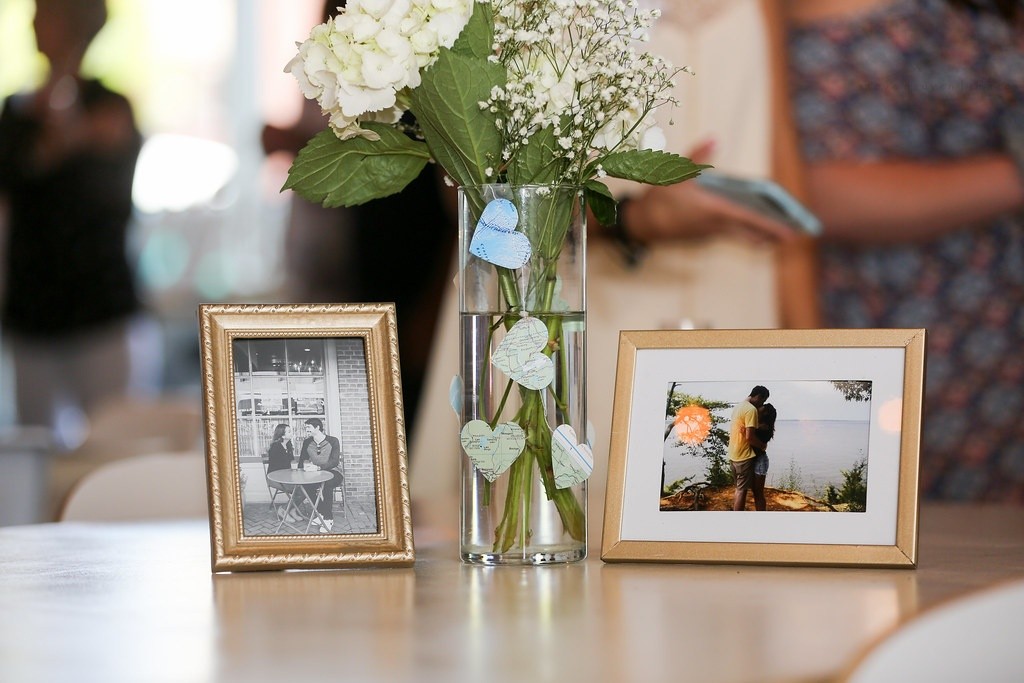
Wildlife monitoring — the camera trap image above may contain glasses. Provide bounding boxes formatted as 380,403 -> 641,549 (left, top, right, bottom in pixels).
317,444 -> 320,455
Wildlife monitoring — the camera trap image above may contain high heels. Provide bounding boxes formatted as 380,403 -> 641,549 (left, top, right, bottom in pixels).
277,506 -> 295,522
289,507 -> 303,521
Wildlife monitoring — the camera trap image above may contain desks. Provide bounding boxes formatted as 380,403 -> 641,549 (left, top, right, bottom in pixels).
0,448 -> 1024,683
266,469 -> 334,535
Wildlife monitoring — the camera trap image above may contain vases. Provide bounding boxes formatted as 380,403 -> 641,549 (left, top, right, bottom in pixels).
451,183 -> 591,573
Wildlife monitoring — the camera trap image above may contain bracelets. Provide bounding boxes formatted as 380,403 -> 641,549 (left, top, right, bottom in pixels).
603,196 -> 647,268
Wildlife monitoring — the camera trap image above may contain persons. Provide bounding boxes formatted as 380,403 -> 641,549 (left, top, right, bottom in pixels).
298,417 -> 340,533
728,386 -> 777,511
784,0 -> 1024,504
408,0 -> 822,501
267,424 -> 306,523
0,0 -> 143,430
260,0 -> 457,434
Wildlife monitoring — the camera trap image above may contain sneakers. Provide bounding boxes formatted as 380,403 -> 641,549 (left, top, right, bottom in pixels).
319,519 -> 333,533
311,513 -> 324,525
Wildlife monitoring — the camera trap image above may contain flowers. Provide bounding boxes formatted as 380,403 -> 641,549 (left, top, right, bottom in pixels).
280,0 -> 714,555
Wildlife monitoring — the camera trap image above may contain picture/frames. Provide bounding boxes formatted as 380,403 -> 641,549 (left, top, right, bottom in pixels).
600,329 -> 931,573
197,299 -> 417,573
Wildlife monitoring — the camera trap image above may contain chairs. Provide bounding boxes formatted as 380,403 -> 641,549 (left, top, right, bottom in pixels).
261,453 -> 307,522
329,452 -> 347,519
